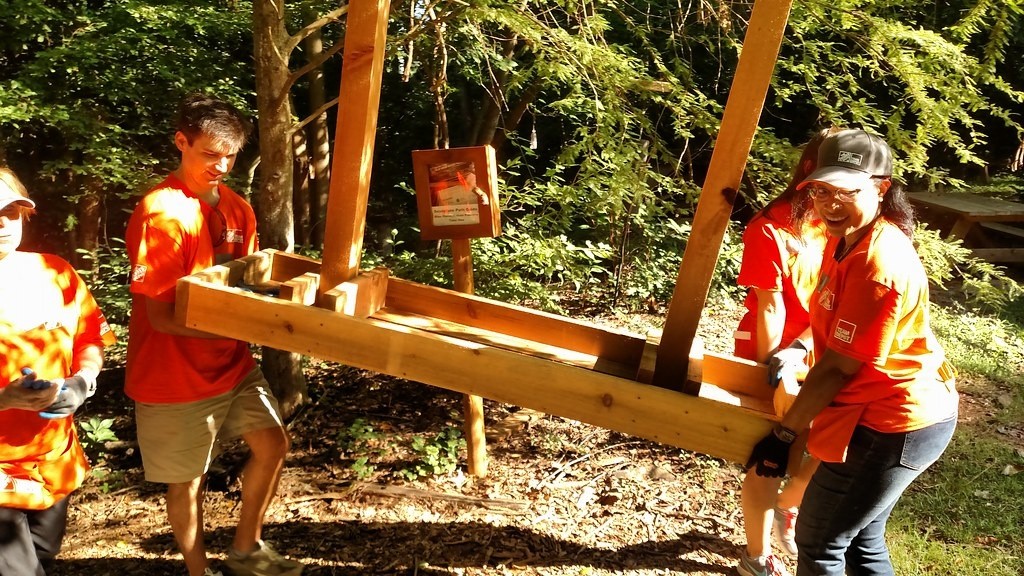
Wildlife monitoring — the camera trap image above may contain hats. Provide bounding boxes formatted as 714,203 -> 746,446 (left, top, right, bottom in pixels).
796,127 -> 895,191
0,177 -> 36,216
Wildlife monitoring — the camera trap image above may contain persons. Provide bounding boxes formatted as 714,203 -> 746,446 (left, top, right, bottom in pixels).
0,167 -> 119,576
123,92 -> 310,576
744,127 -> 959,576
733,127 -> 862,576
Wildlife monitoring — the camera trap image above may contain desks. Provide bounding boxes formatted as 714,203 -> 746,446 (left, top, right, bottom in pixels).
905,192 -> 1024,262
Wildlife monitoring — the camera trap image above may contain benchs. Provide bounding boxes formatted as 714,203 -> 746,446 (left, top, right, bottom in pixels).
981,221 -> 1024,237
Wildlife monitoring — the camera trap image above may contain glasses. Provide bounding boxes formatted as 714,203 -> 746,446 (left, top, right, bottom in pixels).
806,185 -> 876,202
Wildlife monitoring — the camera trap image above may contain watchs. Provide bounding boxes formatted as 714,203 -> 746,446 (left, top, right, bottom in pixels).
774,424 -> 797,444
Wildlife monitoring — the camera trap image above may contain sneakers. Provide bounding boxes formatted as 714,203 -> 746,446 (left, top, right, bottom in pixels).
226,539 -> 306,576
773,506 -> 798,558
737,547 -> 794,576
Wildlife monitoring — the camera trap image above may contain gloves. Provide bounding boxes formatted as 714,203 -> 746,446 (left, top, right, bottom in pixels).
765,338 -> 809,389
39,372 -> 91,419
20,368 -> 66,391
745,423 -> 799,480
